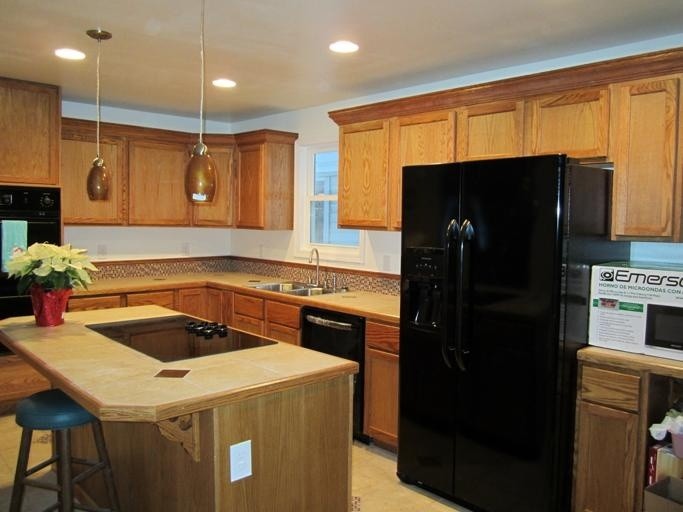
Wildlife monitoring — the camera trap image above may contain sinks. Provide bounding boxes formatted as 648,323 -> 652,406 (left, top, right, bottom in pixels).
256,283 -> 306,292
283,289 -> 331,296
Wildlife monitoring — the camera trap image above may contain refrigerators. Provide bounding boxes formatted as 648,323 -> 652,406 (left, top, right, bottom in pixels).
395,153 -> 614,512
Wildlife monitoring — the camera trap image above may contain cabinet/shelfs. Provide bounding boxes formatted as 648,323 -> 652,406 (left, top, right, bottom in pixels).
327,87 -> 457,232
364,311 -> 400,456
61,117 -> 194,227
235,287 -> 301,346
570,346 -> 683,512
0,75 -> 61,188
176,283 -> 206,318
611,47 -> 683,243
193,129 -> 298,230
458,59 -> 611,164
206,282 -> 234,328
66,284 -> 175,312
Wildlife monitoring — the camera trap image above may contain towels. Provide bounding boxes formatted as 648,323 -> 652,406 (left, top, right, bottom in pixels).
1,220 -> 28,273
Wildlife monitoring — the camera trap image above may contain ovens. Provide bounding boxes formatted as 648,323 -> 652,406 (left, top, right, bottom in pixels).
0,185 -> 61,357
298,306 -> 364,444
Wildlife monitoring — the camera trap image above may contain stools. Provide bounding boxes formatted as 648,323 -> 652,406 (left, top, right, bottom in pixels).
10,388 -> 120,512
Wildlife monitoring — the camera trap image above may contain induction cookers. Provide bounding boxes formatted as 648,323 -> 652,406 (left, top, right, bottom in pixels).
86,313 -> 278,364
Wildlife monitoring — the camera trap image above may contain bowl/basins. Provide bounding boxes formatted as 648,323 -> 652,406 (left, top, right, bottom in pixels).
669,432 -> 683,459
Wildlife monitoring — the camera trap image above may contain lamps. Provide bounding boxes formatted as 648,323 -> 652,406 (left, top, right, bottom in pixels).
86,28 -> 111,201
183,4 -> 219,204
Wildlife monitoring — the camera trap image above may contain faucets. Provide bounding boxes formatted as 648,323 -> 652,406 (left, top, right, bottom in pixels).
309,247 -> 319,285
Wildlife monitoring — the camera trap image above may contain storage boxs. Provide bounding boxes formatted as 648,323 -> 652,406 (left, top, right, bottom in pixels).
644,475 -> 683,512
588,261 -> 683,361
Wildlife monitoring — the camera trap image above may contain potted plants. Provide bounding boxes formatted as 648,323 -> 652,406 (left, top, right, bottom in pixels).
7,240 -> 100,327
